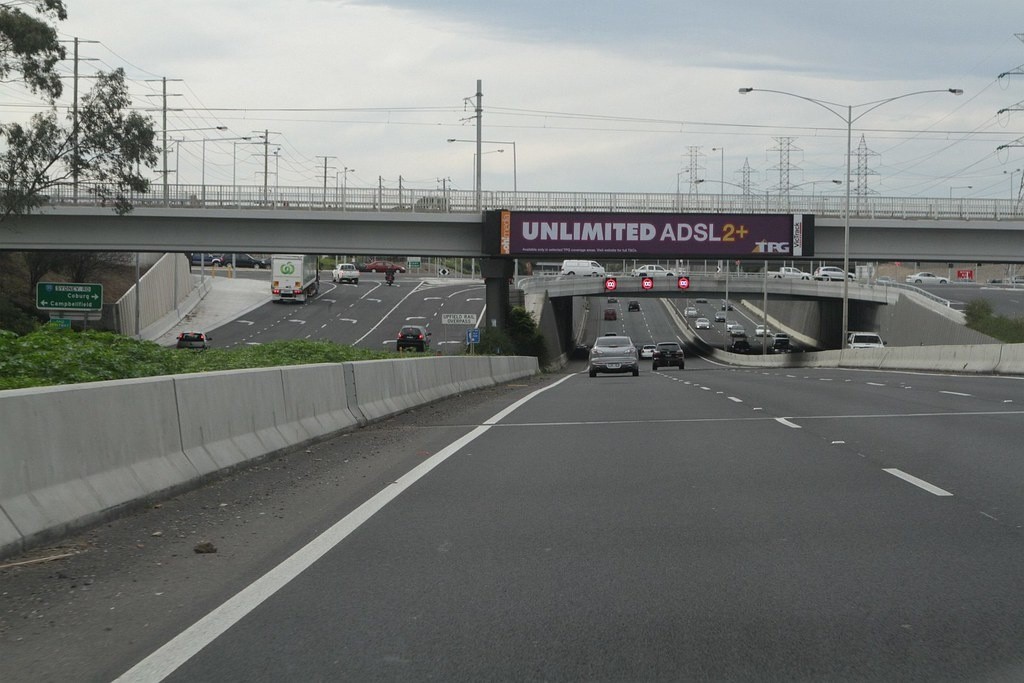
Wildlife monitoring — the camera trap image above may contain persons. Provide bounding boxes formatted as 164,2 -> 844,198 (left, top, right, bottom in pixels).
385,266 -> 394,283
